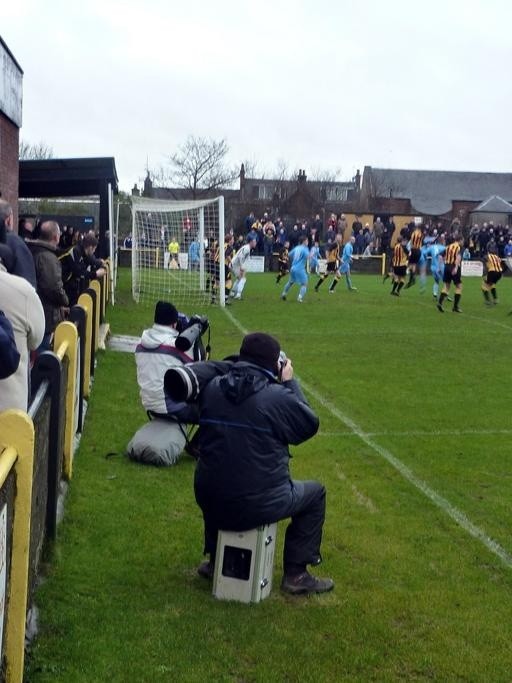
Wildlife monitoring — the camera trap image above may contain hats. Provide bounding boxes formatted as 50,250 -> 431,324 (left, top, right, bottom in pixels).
154,300 -> 179,325
239,332 -> 280,376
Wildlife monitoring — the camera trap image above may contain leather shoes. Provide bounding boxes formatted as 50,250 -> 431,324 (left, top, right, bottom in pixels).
184,441 -> 200,459
197,559 -> 214,579
279,569 -> 335,594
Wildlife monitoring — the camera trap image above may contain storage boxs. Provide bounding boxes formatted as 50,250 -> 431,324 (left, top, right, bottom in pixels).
212,523 -> 277,604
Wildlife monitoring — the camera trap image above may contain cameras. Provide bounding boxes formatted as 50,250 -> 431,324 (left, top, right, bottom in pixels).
276,351 -> 287,378
174,312 -> 209,351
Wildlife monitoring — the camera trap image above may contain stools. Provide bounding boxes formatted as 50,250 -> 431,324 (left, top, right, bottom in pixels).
146,409 -> 196,445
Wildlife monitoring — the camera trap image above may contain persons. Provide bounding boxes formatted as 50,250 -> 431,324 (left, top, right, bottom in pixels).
135,302 -> 203,457
125,203 -> 511,315
0,201 -> 107,433
191,332 -> 335,596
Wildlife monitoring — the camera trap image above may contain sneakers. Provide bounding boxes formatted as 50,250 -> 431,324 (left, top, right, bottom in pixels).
391,290 -> 400,296
282,293 -> 287,301
436,302 -> 444,312
297,294 -> 304,303
447,296 -> 453,302
453,307 -> 463,313
350,286 -> 357,291
432,293 -> 438,303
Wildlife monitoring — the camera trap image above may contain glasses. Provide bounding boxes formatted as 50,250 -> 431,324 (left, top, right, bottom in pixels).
57,231 -> 62,235
